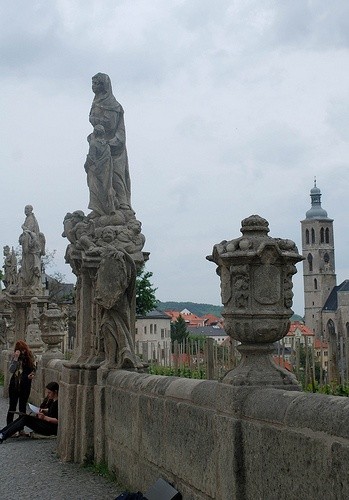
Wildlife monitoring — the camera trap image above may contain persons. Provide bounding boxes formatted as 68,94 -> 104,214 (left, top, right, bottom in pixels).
87,73 -> 131,209
0,382 -> 59,444
5,342 -> 36,438
21,205 -> 40,276
62,211 -> 95,250
97,227 -> 116,248
85,125 -> 113,219
2,246 -> 14,284
121,216 -> 141,240
